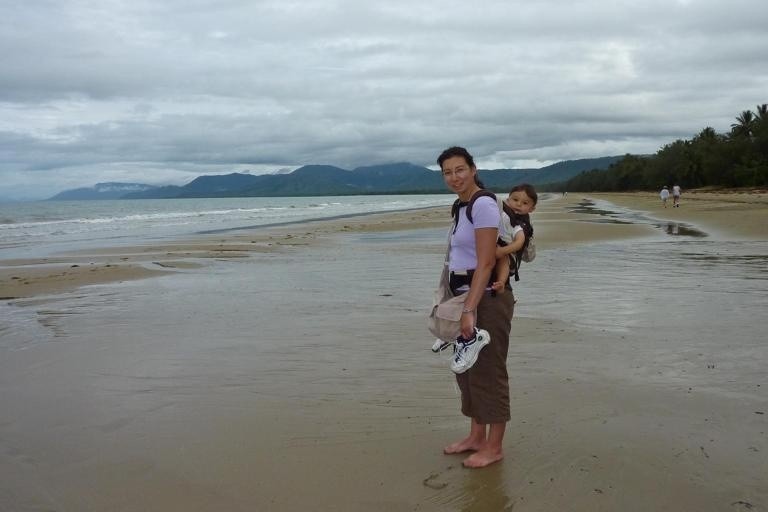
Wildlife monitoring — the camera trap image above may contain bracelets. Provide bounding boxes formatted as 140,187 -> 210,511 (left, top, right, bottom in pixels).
462,308 -> 475,313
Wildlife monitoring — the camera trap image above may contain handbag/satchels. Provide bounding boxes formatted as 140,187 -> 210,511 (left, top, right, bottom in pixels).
427,265 -> 477,342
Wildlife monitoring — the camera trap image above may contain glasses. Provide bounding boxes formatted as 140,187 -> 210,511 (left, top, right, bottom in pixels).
443,165 -> 470,177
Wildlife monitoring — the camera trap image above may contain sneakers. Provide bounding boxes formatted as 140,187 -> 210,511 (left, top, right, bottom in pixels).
448,326 -> 491,375
431,339 -> 452,353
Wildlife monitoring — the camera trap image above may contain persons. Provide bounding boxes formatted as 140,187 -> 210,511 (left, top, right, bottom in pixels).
672,182 -> 681,208
659,185 -> 669,209
491,183 -> 537,295
434,146 -> 517,471
